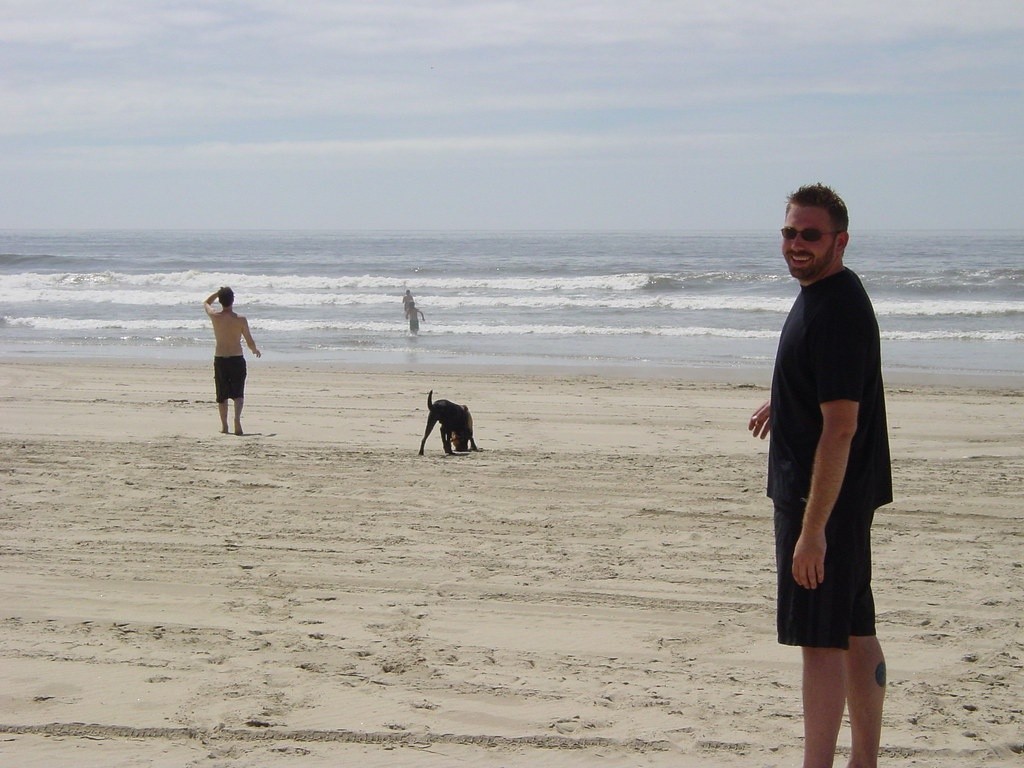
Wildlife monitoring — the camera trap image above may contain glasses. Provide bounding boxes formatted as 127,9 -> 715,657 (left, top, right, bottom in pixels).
780,226 -> 835,242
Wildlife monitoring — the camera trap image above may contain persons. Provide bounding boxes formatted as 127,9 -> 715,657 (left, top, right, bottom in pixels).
745,183 -> 895,768
402,289 -> 414,319
405,301 -> 425,335
201,287 -> 262,435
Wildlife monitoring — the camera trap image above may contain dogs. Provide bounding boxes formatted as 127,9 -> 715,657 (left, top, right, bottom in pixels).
417,388 -> 481,459
